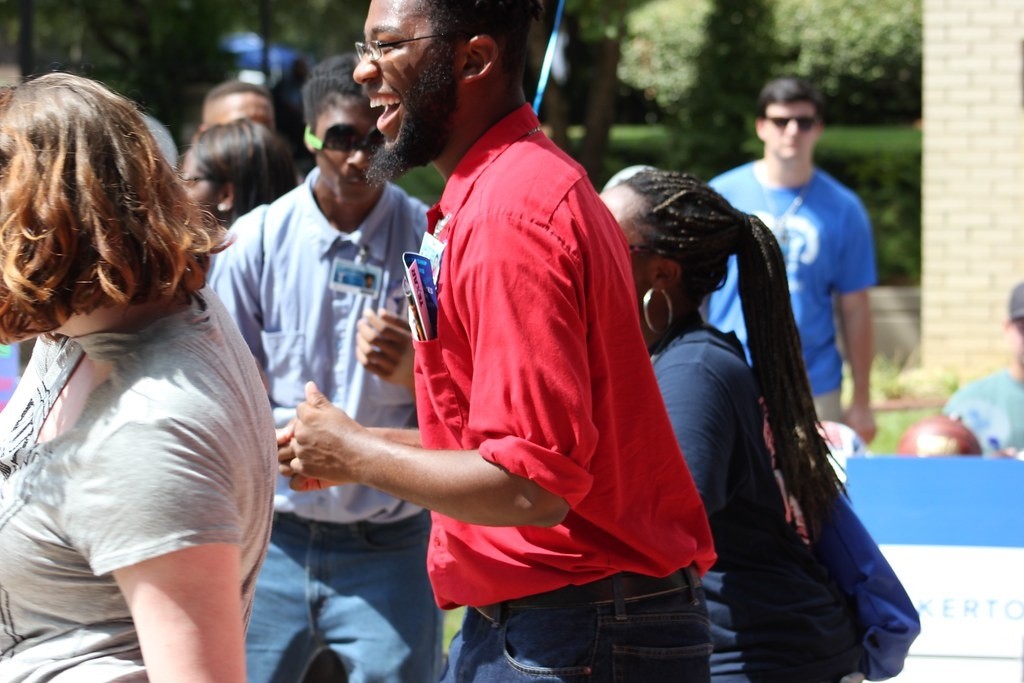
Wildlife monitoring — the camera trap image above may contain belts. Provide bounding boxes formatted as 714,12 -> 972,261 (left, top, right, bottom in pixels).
470,563 -> 704,623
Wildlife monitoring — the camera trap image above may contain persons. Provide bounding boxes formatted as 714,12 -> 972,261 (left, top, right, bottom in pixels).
278,0 -> 717,681
198,82 -> 275,131
946,278 -> 1023,459
594,169 -> 922,683
180,120 -> 298,228
700,80 -> 878,454
2,73 -> 277,681
209,52 -> 442,682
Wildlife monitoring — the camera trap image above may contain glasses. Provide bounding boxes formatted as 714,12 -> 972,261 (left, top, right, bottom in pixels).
355,30 -> 471,61
178,175 -> 213,187
764,115 -> 820,132
304,124 -> 385,155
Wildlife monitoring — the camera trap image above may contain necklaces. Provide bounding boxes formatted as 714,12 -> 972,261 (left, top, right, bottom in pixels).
758,160 -> 812,228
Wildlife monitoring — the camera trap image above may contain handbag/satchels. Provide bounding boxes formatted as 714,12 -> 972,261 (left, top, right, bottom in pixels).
728,331 -> 922,682
